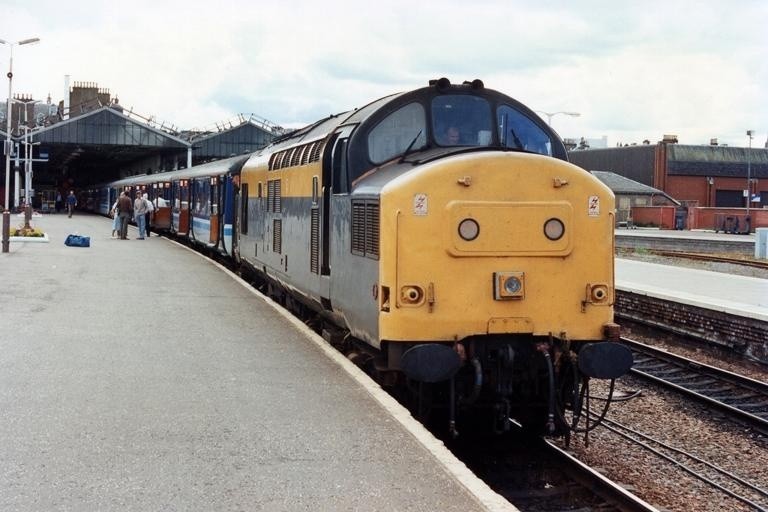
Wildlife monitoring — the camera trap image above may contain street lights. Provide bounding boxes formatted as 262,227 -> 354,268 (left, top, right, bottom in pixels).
532,107 -> 582,126
745,129 -> 753,215
0,35 -> 40,256
9,94 -> 46,239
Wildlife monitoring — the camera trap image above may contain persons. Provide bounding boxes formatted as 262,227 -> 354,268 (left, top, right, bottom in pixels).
111,191 -> 160,240
66,190 -> 78,218
152,193 -> 166,207
56,191 -> 63,213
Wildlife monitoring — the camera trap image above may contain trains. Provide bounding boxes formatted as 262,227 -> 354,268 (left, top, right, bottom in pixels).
78,73 -> 634,469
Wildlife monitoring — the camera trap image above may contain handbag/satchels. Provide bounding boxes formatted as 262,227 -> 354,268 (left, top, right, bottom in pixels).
64,234 -> 91,247
